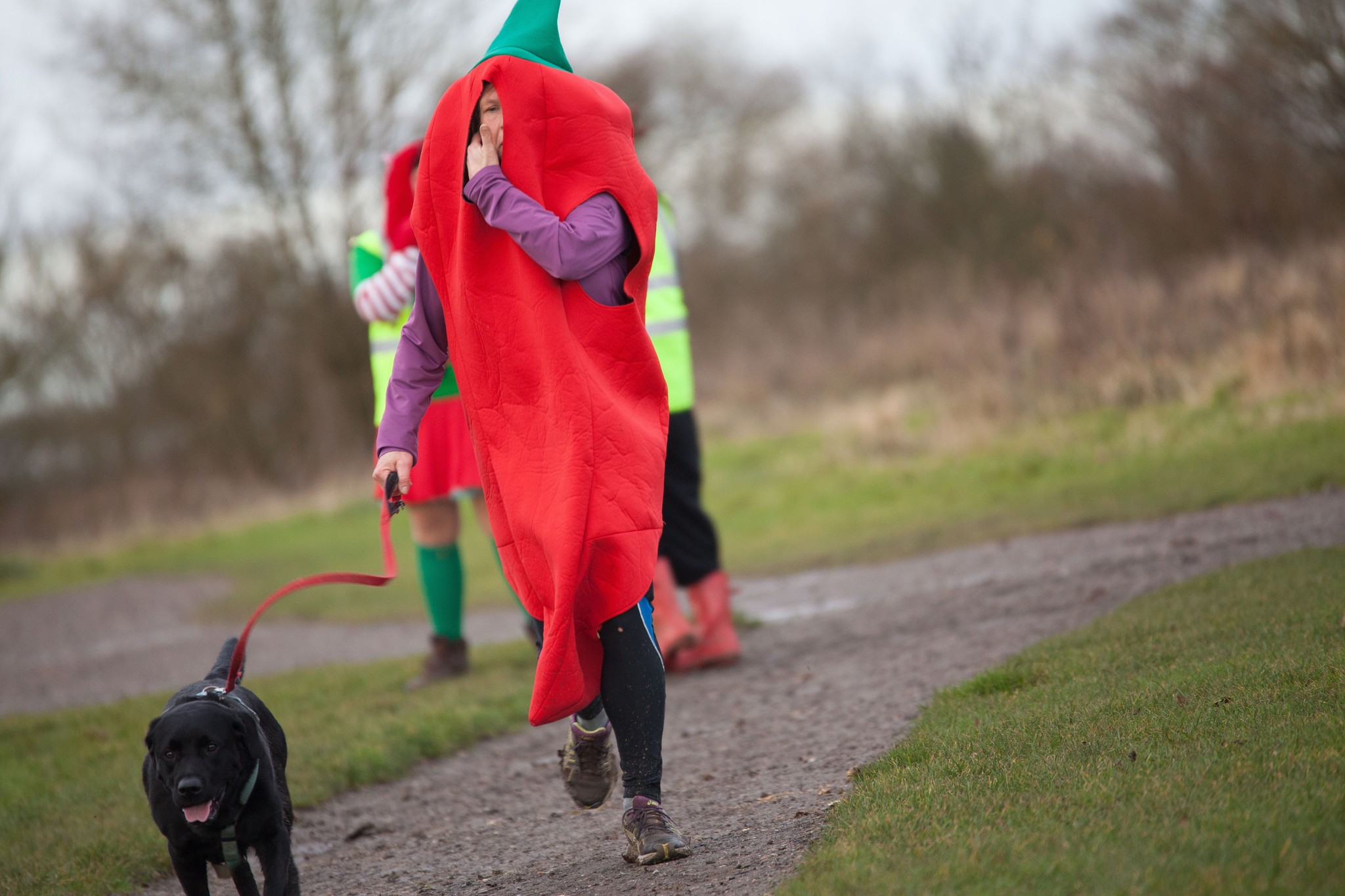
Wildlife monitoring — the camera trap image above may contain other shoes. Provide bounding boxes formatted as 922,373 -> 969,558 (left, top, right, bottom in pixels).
405,634 -> 469,694
649,555 -> 741,672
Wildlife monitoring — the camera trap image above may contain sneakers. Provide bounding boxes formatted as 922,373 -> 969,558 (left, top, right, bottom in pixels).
557,712 -> 619,810
621,795 -> 692,866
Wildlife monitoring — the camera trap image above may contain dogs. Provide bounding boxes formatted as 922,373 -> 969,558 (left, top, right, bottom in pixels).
142,638 -> 301,896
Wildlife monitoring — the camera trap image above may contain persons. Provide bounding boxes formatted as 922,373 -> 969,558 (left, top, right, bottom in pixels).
629,186 -> 741,679
368,63 -> 694,871
345,137 -> 562,678
